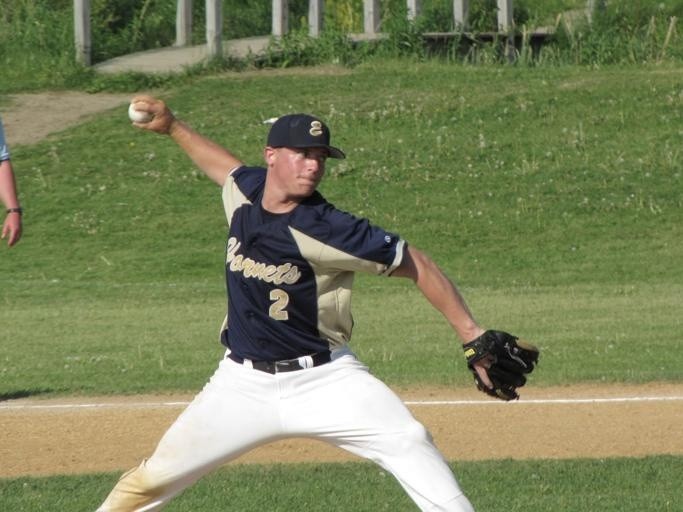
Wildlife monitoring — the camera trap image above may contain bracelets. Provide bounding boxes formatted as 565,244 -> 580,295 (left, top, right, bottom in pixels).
4,207 -> 22,217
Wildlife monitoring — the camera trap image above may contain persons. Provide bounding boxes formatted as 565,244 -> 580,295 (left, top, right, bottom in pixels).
93,92 -> 538,511
0,114 -> 22,249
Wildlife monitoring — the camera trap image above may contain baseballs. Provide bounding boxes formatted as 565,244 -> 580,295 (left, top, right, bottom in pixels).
128,99 -> 152,123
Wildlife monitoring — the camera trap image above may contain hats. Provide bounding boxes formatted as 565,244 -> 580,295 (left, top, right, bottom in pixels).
264,113 -> 347,163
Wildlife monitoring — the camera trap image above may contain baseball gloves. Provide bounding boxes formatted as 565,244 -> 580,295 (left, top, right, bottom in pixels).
462,331 -> 539,400
